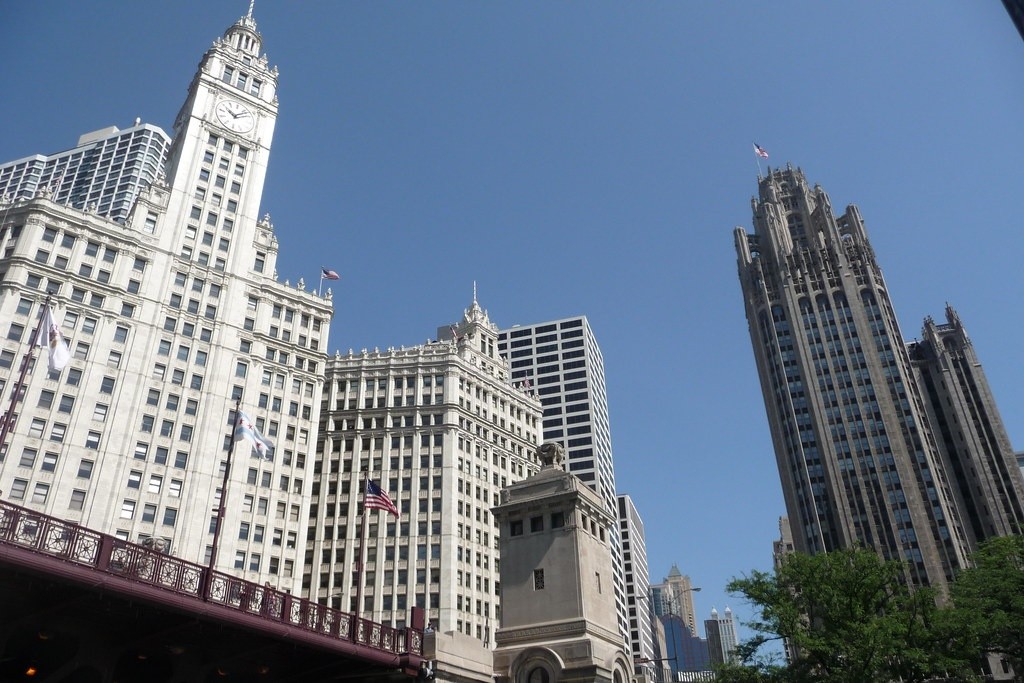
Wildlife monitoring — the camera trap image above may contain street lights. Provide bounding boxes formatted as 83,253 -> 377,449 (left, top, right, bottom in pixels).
665,588 -> 703,671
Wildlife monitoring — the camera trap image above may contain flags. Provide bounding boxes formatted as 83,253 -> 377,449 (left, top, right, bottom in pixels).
754,142 -> 768,158
57,169 -> 63,185
234,409 -> 273,459
450,327 -> 456,337
321,268 -> 340,280
36,304 -> 71,373
365,477 -> 399,519
525,379 -> 530,389
